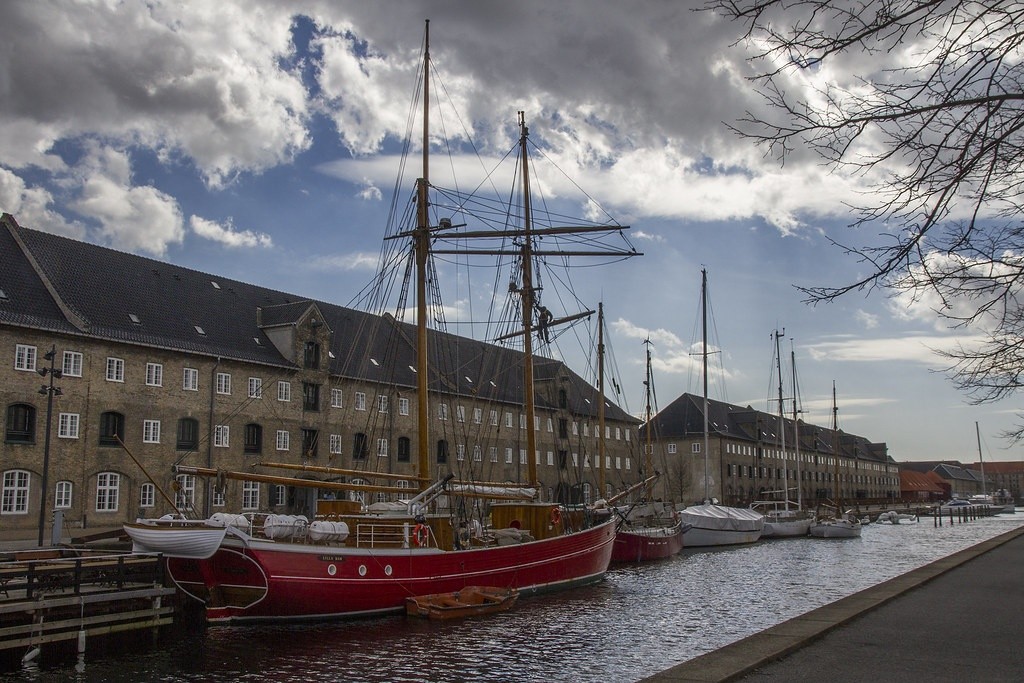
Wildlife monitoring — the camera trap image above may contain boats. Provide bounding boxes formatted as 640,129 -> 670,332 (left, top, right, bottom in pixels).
405,585 -> 521,621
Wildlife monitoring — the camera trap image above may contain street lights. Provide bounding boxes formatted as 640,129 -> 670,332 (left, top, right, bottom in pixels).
37,343 -> 62,546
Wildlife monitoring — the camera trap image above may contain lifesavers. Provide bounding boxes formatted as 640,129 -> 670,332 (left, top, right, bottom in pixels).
412,523 -> 428,546
551,508 -> 561,524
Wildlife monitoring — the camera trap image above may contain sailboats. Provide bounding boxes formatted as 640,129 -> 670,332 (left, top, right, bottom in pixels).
928,422 -> 1006,515
119,16 -> 618,622
747,327 -> 817,536
810,379 -> 862,538
676,269 -> 763,548
610,337 -> 684,560
